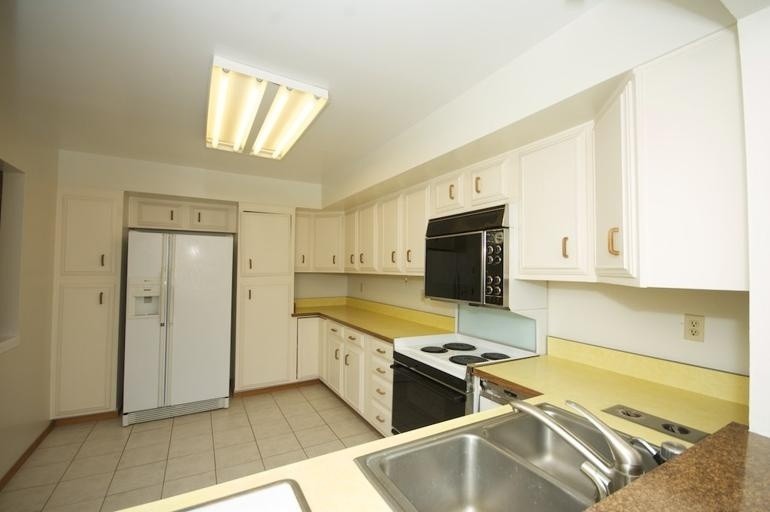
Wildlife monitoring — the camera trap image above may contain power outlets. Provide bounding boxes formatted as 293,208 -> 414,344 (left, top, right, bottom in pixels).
684,314 -> 705,342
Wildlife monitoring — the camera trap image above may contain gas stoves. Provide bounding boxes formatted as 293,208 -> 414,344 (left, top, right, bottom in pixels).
392,335 -> 540,395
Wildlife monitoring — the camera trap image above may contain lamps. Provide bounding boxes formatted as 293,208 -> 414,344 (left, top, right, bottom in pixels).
205,56 -> 329,161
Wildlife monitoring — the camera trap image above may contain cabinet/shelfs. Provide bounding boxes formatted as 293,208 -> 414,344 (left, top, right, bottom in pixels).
240,207 -> 292,277
58,278 -> 117,413
125,192 -> 238,235
235,277 -> 293,392
323,319 -> 394,437
294,210 -> 315,273
521,119 -> 596,283
57,193 -> 118,277
596,81 -> 641,287
378,180 -> 427,277
430,149 -> 511,216
344,201 -> 378,274
315,210 -> 344,273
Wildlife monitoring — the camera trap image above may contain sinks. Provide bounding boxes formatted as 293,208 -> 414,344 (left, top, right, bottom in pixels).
484,401 -> 676,503
353,431 -> 590,512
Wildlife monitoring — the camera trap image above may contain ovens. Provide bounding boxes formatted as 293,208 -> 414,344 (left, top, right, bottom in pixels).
390,358 -> 466,437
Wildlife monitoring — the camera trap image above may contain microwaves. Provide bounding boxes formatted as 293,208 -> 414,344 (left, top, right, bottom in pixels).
423,228 -> 509,309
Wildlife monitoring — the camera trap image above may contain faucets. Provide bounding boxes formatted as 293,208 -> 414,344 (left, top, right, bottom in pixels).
629,437 -> 685,467
508,399 -> 644,504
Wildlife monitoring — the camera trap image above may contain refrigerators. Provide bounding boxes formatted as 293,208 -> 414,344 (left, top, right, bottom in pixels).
119,227 -> 234,429
